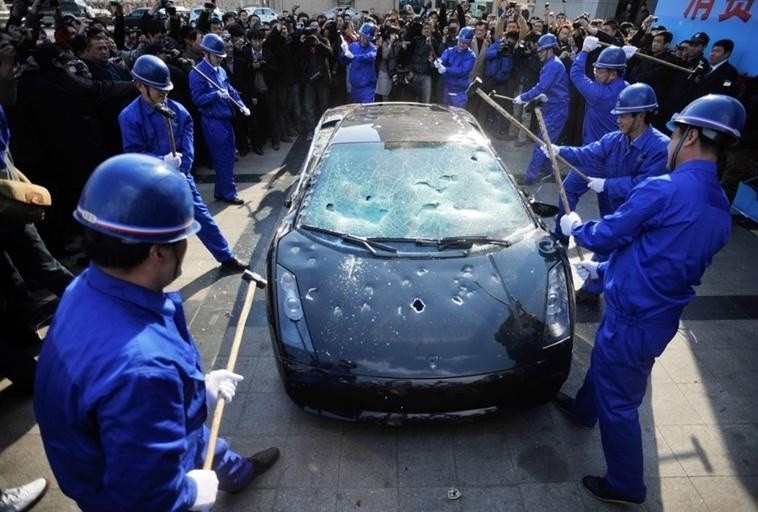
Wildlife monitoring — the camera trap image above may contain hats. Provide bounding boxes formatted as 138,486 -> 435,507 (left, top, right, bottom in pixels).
649,25 -> 667,31
691,31 -> 710,42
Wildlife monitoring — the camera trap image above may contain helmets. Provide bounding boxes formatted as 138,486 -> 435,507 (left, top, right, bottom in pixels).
129,53 -> 174,94
591,46 -> 628,69
534,32 -> 558,52
666,93 -> 746,139
455,26 -> 475,44
73,150 -> 202,246
607,83 -> 659,117
199,33 -> 228,60
358,21 -> 376,40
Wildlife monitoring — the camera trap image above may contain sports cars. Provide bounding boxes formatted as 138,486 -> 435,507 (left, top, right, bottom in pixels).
259,94 -> 579,441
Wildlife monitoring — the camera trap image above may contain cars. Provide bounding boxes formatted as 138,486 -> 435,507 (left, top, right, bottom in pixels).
320,4 -> 361,21
0,0 -> 282,32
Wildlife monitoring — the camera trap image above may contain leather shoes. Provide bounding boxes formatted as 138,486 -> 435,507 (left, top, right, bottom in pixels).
240,137 -> 316,157
214,192 -> 246,208
226,445 -> 280,496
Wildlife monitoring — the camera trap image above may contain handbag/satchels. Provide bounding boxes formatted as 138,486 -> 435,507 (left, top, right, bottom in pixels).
0,177 -> 52,223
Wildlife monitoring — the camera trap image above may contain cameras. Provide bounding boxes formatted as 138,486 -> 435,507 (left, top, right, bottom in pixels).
278,16 -> 290,22
71,62 -> 85,71
519,43 -> 531,53
158,0 -> 177,16
503,44 -> 513,53
509,2 -> 516,7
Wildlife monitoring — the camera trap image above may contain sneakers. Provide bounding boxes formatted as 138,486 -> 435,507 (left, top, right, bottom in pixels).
513,139 -> 527,148
551,391 -> 597,431
218,260 -> 252,271
517,179 -> 537,186
581,475 -> 647,507
541,173 -> 555,183
0,478 -> 49,512
573,287 -> 601,305
496,133 -> 516,140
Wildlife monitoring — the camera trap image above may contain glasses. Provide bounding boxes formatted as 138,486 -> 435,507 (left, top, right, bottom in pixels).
593,68 -> 612,74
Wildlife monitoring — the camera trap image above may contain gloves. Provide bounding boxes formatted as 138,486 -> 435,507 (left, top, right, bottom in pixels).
573,260 -> 600,282
512,95 -> 523,106
182,468 -> 219,512
433,59 -> 447,75
340,42 -> 353,61
239,105 -> 252,119
558,211 -> 583,238
587,176 -> 606,195
164,149 -> 184,169
540,141 -> 560,162
216,88 -> 230,101
204,367 -> 245,408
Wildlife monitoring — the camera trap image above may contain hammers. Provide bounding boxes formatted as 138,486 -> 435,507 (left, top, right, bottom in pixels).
154,102 -> 176,157
597,41 -> 704,81
465,76 -> 592,182
202,268 -> 268,470
488,90 -> 528,103
523,94 -> 591,283
425,37 -> 440,63
178,57 -> 244,113
337,30 -> 348,53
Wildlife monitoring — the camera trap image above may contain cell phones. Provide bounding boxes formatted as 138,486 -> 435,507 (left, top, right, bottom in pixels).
205,3 -> 216,9
477,4 -> 485,10
650,16 -> 659,23
